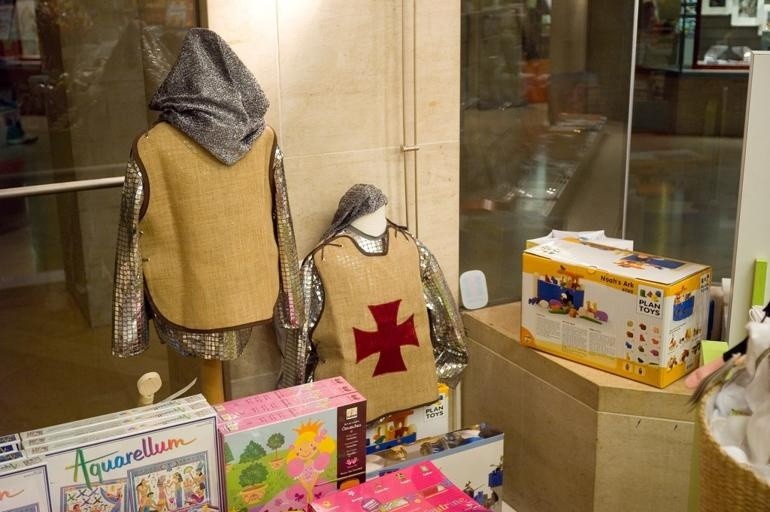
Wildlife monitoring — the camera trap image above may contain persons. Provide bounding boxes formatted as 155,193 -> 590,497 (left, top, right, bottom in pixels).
113,29 -> 303,361
276,183 -> 470,428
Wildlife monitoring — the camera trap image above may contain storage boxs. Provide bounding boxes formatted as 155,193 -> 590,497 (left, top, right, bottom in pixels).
520,229 -> 712,389
369,381 -> 449,450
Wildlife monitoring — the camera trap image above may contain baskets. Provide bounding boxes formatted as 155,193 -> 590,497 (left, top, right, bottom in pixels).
691,360 -> 770,512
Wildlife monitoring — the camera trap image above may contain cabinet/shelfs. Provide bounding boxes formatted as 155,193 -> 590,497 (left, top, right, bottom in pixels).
632,62 -> 754,136
467,299 -> 720,512
